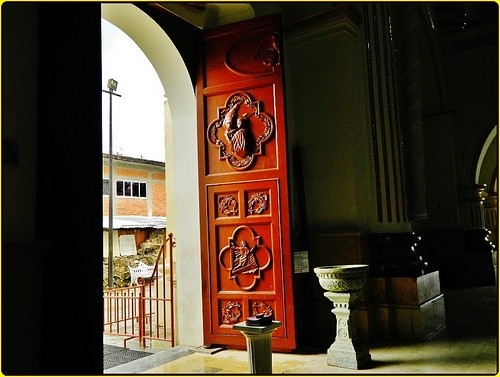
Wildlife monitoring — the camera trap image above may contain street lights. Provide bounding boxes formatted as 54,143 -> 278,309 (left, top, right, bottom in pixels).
102,78 -> 120,289
